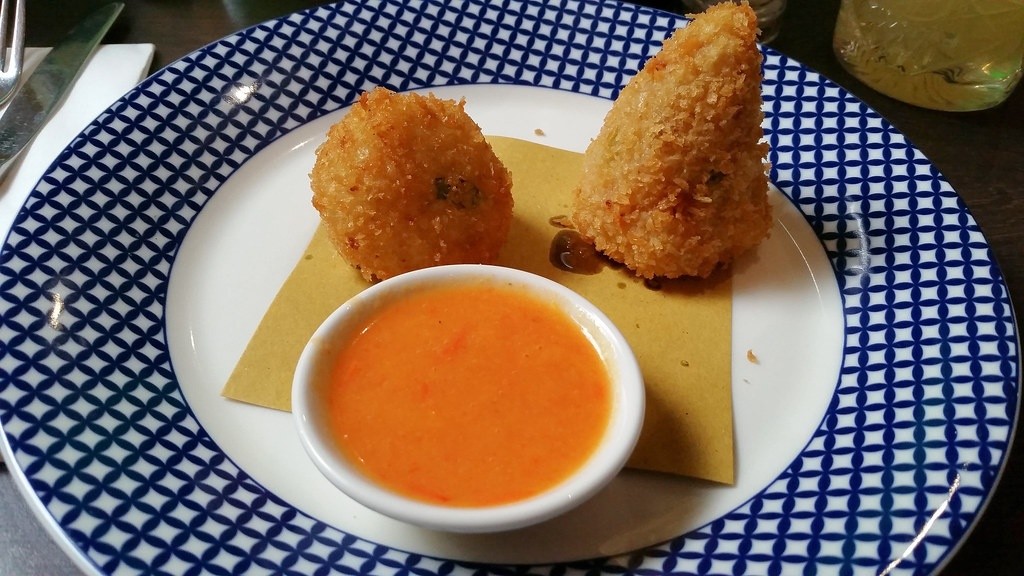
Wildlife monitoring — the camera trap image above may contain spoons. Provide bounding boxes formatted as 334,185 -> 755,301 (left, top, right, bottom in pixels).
0,0 -> 26,107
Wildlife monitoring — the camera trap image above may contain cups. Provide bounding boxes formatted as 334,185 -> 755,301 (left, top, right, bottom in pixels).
696,0 -> 786,46
833,0 -> 1024,113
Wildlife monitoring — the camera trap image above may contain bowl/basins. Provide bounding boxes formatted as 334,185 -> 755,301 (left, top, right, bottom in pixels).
290,263 -> 646,536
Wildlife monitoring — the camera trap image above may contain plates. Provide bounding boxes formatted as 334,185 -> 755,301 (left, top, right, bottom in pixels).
0,0 -> 1024,576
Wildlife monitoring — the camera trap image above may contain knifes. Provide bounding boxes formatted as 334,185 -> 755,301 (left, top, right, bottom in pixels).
0,0 -> 125,186
